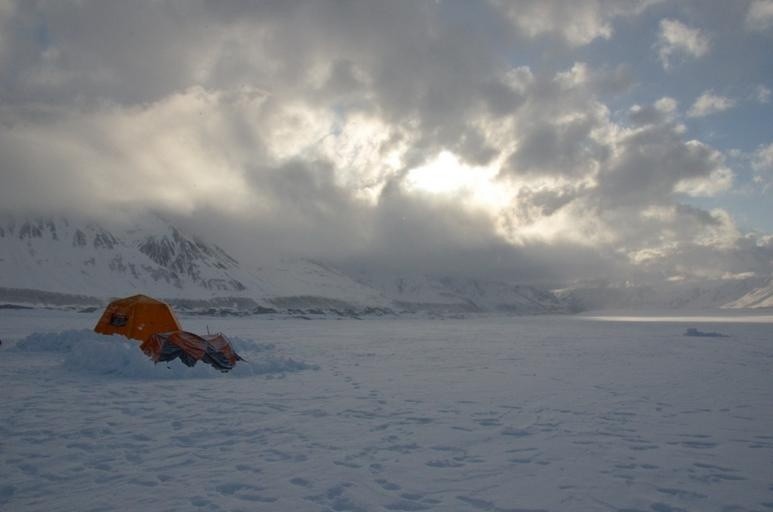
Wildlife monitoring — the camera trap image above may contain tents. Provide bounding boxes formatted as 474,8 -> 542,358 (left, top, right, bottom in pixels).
95,294 -> 182,343
139,329 -> 249,374
